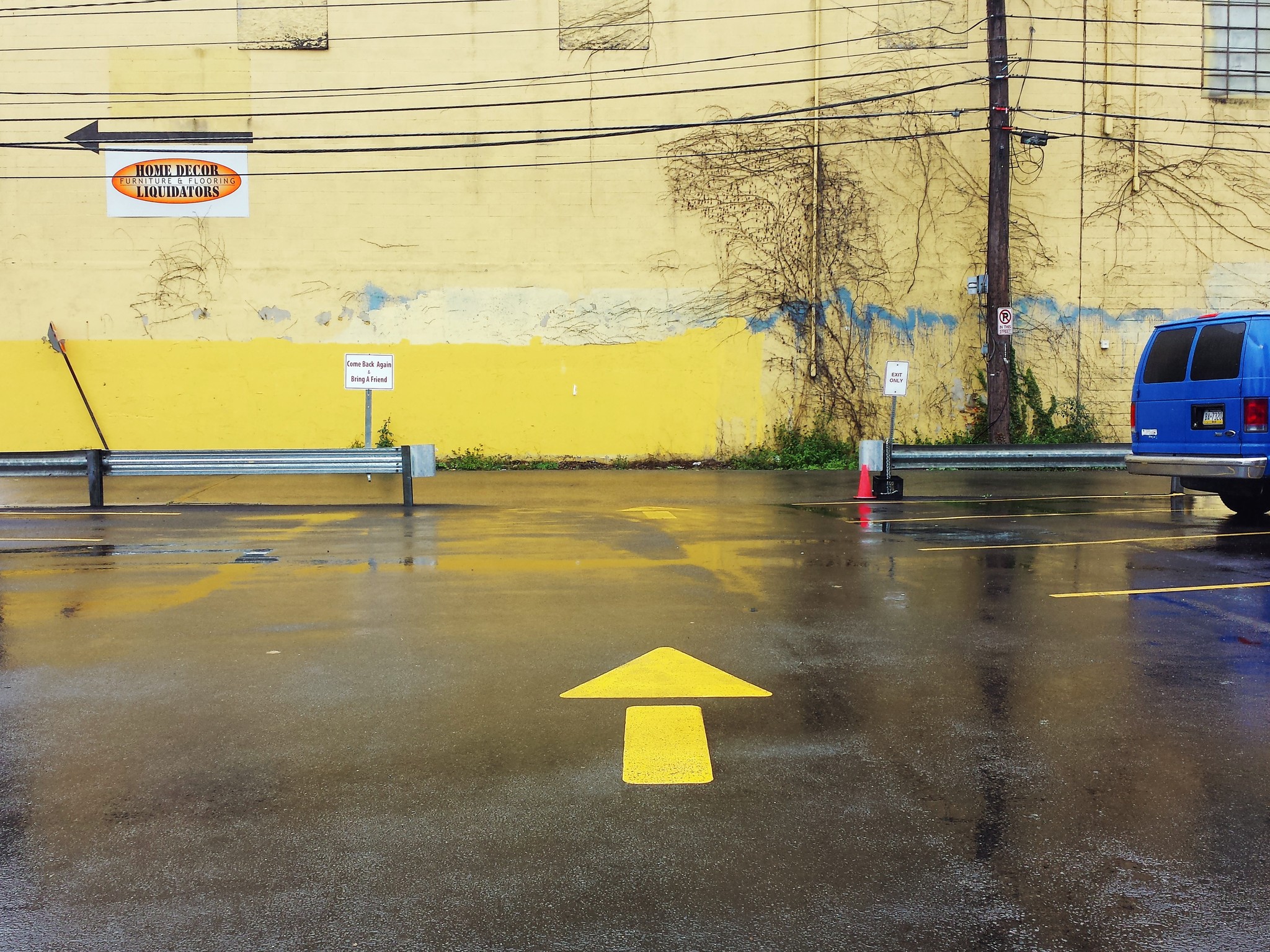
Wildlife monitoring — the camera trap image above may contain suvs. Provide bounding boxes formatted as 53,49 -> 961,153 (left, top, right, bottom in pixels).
1124,309 -> 1269,526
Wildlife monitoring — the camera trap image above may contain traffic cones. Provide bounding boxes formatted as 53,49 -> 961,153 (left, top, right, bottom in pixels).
852,464 -> 876,499
